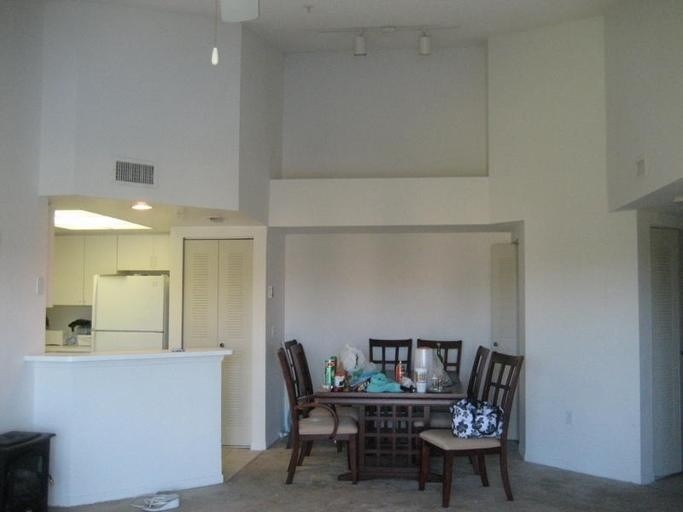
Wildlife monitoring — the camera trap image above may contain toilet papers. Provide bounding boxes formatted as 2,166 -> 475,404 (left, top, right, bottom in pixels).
415,347 -> 442,388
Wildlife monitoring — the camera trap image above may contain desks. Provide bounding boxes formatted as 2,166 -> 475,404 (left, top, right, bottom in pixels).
314,370 -> 469,481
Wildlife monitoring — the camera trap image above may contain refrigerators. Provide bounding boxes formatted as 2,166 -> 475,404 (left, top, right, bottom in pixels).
90,274 -> 168,351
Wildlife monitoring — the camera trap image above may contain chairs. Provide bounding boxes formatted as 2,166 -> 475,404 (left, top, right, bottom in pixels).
277,336 -> 359,487
414,339 -> 463,414
412,344 -> 490,468
418,350 -> 524,508
367,338 -> 411,445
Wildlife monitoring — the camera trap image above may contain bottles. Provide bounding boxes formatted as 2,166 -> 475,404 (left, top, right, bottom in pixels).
435,342 -> 444,363
394,359 -> 405,384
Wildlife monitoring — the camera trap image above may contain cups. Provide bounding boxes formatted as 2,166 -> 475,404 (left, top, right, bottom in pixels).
416,382 -> 426,393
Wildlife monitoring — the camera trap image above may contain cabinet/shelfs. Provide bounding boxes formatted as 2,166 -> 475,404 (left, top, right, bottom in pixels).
53,235 -> 117,306
117,234 -> 170,272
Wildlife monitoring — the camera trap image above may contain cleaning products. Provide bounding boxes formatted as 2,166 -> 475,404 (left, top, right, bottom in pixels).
396,359 -> 406,381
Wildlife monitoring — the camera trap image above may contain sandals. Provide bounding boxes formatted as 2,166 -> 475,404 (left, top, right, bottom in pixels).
129,492 -> 180,512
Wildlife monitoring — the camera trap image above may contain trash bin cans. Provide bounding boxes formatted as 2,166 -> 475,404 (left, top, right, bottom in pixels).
0,431 -> 56,512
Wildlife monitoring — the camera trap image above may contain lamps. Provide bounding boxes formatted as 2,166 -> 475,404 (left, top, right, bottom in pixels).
211,0 -> 219,66
320,25 -> 463,56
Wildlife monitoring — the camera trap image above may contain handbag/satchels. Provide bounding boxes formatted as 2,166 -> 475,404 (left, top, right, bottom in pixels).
447,399 -> 505,441
